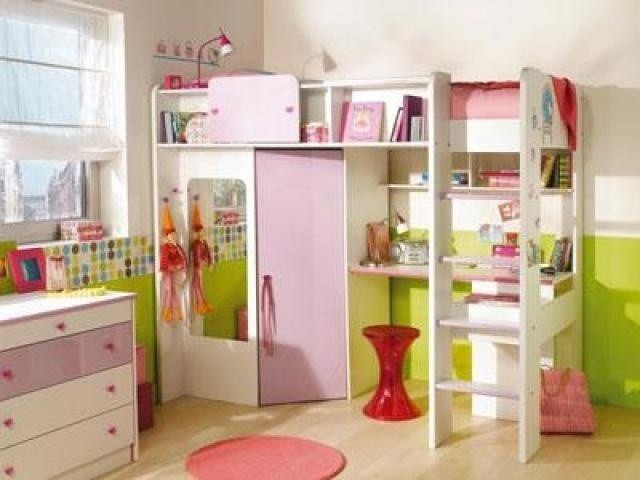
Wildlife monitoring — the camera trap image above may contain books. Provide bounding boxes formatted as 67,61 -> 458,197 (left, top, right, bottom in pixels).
164,112 -> 181,145
340,100 -> 385,140
402,94 -> 423,141
487,151 -> 573,190
409,115 -> 425,142
390,106 -> 404,143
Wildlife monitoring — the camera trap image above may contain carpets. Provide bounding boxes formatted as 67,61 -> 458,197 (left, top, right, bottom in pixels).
185,434 -> 347,480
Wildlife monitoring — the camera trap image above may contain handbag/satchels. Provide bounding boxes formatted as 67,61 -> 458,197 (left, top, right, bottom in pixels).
539,368 -> 594,435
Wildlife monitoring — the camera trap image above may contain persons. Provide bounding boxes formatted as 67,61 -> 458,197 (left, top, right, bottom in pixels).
184,194 -> 215,319
162,195 -> 188,324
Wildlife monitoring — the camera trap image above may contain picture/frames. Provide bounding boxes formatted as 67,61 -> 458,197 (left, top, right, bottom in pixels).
6,248 -> 51,295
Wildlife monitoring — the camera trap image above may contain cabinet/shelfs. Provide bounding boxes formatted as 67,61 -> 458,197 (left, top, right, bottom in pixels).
0,283 -> 141,479
150,66 -> 584,463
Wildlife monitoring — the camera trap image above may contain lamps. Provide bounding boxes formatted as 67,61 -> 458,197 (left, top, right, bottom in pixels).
192,26 -> 234,86
